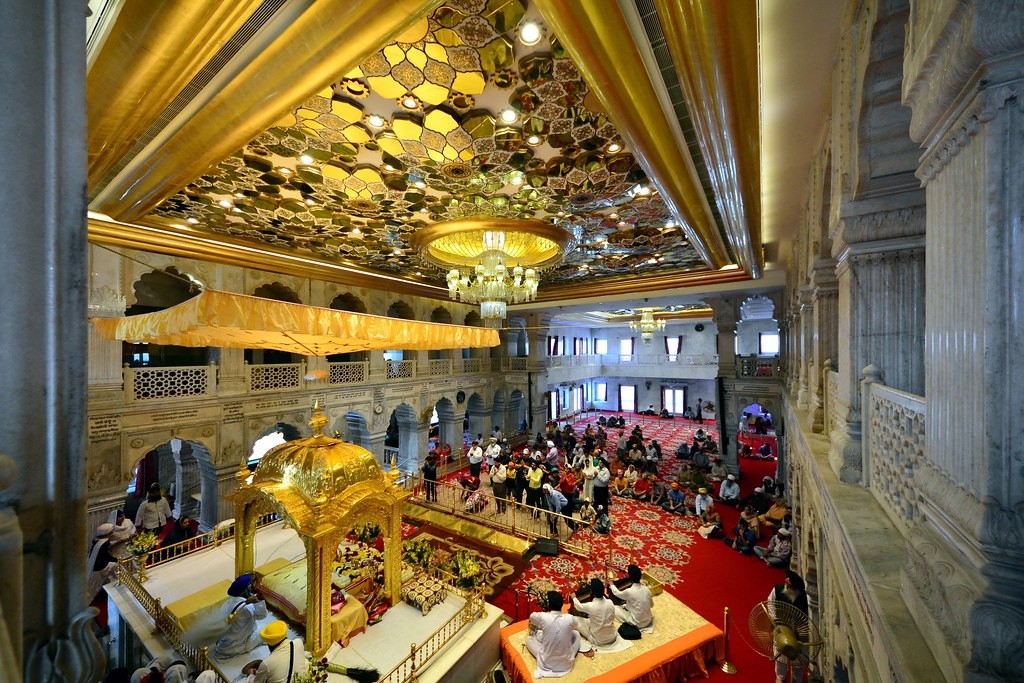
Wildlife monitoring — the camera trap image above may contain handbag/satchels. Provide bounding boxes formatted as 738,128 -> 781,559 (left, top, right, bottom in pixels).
617,622 -> 641,639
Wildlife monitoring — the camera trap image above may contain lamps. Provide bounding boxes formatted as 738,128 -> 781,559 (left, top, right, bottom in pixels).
629,309 -> 666,345
445,234 -> 539,319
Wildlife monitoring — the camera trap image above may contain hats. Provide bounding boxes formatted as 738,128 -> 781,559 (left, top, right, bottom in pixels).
425,456 -> 433,461
96,523 -> 114,537
650,475 -> 658,482
547,591 -> 563,608
591,579 -> 604,598
714,458 -> 721,464
762,475 -> 774,488
670,482 -> 678,488
632,444 -> 638,448
727,474 -> 736,482
551,467 -> 558,474
512,452 -> 518,458
546,441 -> 555,448
698,488 -> 708,495
628,565 -> 642,583
584,496 -> 592,503
226,574 -> 251,597
597,505 -> 604,511
755,487 -> 763,494
494,458 -> 502,462
778,528 -> 789,537
523,448 -> 530,455
786,569 -> 805,591
472,441 -> 478,445
260,620 -> 288,647
618,469 -> 624,475
508,462 -> 514,467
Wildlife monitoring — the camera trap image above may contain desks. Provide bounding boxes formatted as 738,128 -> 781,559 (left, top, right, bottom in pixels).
162,578 -> 236,631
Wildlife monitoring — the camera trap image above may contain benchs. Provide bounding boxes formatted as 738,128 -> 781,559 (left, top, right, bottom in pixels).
255,558 -> 290,580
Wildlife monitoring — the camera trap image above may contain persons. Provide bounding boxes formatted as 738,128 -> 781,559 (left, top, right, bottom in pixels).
215,575 -> 267,660
616,424 -> 662,477
430,421 -> 613,539
421,456 -> 437,502
673,426 -> 729,484
699,504 -> 724,539
646,404 -> 655,416
130,655 -> 215,683
596,414 -> 625,429
737,411 -> 775,461
767,571 -> 808,683
688,488 -> 713,518
684,398 -> 704,424
570,578 -> 617,646
525,591 -> 581,672
247,620 -> 306,683
89,483 -> 195,572
612,464 -> 688,518
608,564 -> 654,628
716,474 -> 792,566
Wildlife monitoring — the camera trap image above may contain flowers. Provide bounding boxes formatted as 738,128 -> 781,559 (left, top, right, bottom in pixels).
403,536 -> 435,567
445,549 -> 480,586
127,530 -> 158,555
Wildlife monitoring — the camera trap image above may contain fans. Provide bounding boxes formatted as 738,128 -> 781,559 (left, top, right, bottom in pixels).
749,600 -> 821,683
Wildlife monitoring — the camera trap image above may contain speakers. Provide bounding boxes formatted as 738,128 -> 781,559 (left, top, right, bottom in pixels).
535,538 -> 559,555
